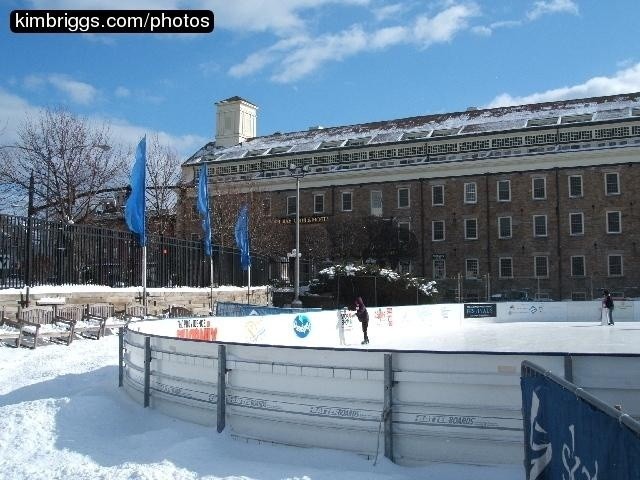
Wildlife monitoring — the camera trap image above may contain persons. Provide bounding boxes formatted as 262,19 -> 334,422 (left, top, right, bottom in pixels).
602,289 -> 614,326
351,296 -> 370,345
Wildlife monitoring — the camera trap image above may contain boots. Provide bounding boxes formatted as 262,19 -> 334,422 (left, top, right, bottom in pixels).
361,340 -> 369,344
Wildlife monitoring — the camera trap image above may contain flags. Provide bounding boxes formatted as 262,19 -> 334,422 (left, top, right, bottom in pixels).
197,162 -> 213,257
235,204 -> 251,271
123,132 -> 146,247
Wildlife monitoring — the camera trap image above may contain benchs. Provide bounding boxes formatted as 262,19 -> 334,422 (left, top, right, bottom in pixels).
610,291 -> 624,301
534,289 -> 553,301
571,287 -> 589,301
1,299 -> 198,350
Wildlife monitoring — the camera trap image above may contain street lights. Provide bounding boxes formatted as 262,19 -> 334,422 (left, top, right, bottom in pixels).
288,163 -> 309,308
46,146 -> 113,221
